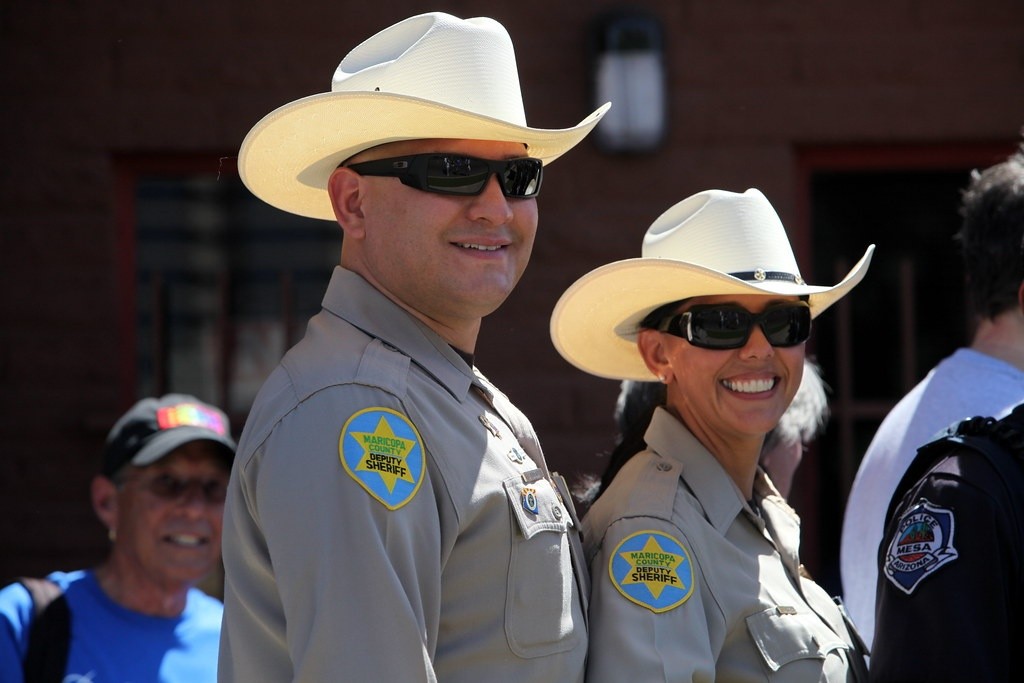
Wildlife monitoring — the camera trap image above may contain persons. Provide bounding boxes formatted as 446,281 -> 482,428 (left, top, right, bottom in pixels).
841,156 -> 1024,667
548,188 -> 876,683
868,401 -> 1024,683
0,394 -> 238,683
218,18 -> 613,683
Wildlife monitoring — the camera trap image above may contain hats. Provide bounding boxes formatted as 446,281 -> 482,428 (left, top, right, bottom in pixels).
549,188 -> 876,381
98,391 -> 237,483
238,11 -> 611,225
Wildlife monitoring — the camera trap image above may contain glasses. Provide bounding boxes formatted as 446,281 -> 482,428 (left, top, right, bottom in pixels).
660,299 -> 813,351
115,471 -> 226,503
350,150 -> 543,200
